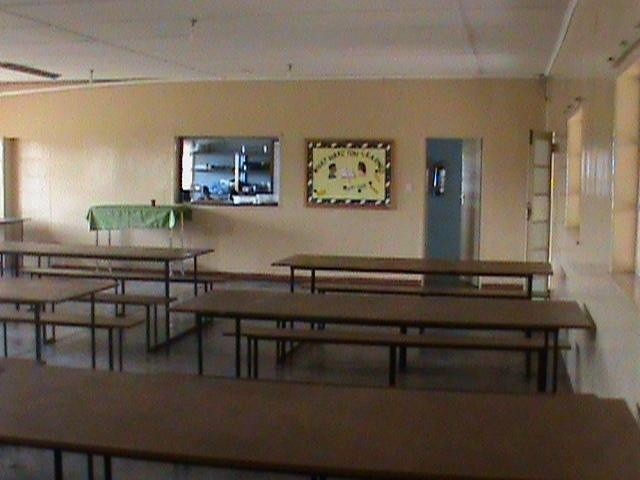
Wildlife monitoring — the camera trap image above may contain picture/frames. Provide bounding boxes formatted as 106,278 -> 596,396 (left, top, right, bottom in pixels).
304,138 -> 391,209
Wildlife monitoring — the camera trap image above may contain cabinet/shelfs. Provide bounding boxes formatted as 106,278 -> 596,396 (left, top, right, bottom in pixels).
190,144 -> 274,192
